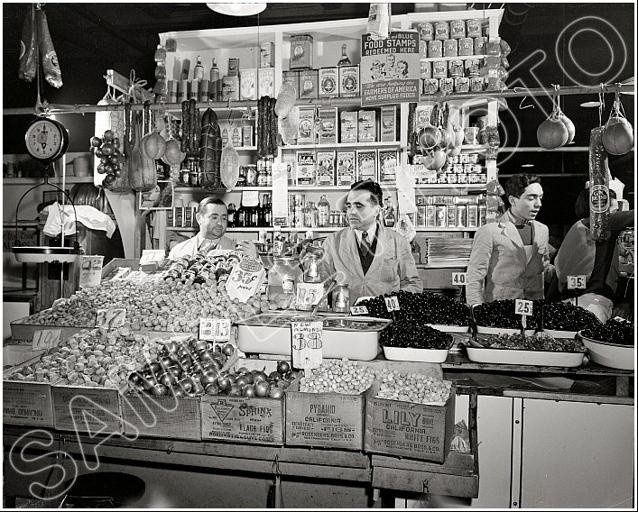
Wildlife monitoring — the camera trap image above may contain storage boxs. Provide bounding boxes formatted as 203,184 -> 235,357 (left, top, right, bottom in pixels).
364,380 -> 456,465
3,353 -> 74,428
52,378 -> 127,438
201,357 -> 292,446
285,376 -> 364,455
120,388 -> 201,440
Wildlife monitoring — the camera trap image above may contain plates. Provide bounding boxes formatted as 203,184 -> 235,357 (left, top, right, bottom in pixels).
465,346 -> 584,368
349,296 -> 469,333
472,304 -> 576,339
381,344 -> 449,364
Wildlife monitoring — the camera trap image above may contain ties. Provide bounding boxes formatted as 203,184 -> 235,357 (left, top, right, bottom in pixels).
360,229 -> 370,256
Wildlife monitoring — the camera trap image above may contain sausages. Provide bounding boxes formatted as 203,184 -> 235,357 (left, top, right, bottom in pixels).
189,98 -> 197,152
180,100 -> 188,152
193,107 -> 201,149
261,95 -> 270,155
206,125 -> 223,191
589,127 -> 611,241
199,107 -> 218,187
256,99 -> 263,159
270,98 -> 277,150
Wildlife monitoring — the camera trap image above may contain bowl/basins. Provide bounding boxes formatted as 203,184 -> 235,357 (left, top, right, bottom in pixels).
233,314 -> 393,362
579,329 -> 634,371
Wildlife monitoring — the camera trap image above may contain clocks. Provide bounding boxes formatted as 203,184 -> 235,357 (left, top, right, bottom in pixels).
24,111 -> 69,162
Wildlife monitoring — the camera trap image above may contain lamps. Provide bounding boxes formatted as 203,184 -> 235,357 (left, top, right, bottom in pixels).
205,3 -> 267,16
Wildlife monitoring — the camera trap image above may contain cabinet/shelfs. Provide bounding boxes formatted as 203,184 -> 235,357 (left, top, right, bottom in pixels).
3,176 -> 134,345
155,9 -> 505,290
393,398 -> 623,507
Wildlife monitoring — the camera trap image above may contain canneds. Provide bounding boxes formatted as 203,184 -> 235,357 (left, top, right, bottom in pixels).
415,18 -> 488,96
411,154 -> 487,184
464,126 -> 480,145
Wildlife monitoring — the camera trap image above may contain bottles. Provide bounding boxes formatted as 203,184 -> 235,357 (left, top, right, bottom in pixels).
257,171 -> 266,186
266,156 -> 274,171
250,206 -> 256,227
334,210 -> 340,224
330,210 -> 334,224
222,128 -> 229,147
263,203 -> 269,227
304,201 -> 312,227
246,165 -> 257,185
232,128 -> 240,147
331,283 -> 350,313
209,56 -> 220,82
256,156 -> 266,171
181,169 -> 189,184
304,254 -> 323,283
187,156 -> 196,172
336,43 -> 352,66
308,201 -> 320,227
269,202 -> 272,227
266,171 -> 272,186
267,256 -> 303,307
237,206 -> 245,227
340,210 -> 347,226
245,207 -> 250,227
194,55 -> 204,83
256,202 -> 263,227
317,194 -> 330,226
189,172 -> 198,185
227,203 -> 237,227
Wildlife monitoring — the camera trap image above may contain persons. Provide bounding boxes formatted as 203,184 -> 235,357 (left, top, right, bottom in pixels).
369,54 -> 409,82
321,180 -> 424,305
168,197 -> 258,262
464,173 -> 550,307
546,187 -> 619,301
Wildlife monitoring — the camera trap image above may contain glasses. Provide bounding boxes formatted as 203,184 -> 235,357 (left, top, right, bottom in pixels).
346,204 -> 365,211
